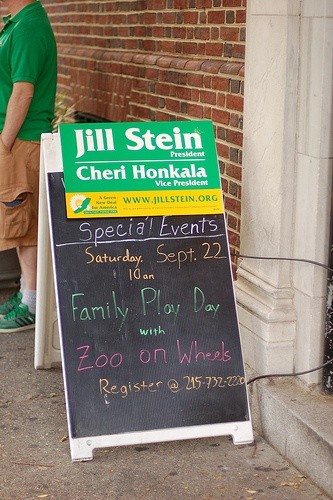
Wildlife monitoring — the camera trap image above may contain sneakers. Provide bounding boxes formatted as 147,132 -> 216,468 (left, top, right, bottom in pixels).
0,291 -> 23,316
1,306 -> 35,334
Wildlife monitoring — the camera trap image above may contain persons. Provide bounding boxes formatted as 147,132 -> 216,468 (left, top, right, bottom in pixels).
0,1 -> 57,334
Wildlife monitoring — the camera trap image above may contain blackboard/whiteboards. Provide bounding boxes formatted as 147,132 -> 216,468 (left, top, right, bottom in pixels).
39,132 -> 252,450
